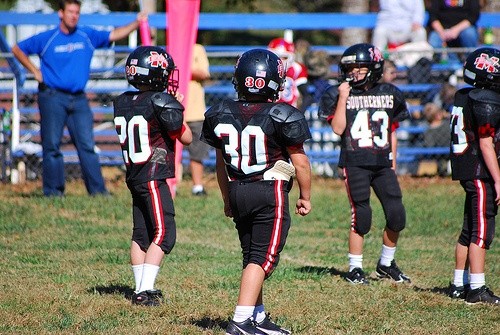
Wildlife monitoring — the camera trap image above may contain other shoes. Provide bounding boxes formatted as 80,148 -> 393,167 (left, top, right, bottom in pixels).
191,186 -> 207,197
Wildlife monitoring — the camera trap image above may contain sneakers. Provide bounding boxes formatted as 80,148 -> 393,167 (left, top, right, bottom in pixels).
375,258 -> 411,285
255,312 -> 292,335
132,289 -> 167,306
465,285 -> 500,305
346,267 -> 373,286
447,281 -> 470,302
225,315 -> 268,335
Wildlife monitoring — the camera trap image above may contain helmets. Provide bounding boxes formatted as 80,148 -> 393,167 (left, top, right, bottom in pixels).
337,43 -> 384,94
267,37 -> 295,72
125,46 -> 179,96
463,48 -> 500,91
232,49 -> 286,102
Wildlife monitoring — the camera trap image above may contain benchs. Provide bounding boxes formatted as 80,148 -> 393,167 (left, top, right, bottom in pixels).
0,11 -> 500,178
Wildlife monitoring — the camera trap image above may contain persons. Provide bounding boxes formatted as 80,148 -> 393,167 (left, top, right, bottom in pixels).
447,46 -> 499,304
112,45 -> 192,308
204,48 -> 312,335
254,0 -> 483,147
318,42 -> 412,287
11,1 -> 148,197
175,43 -> 211,196
